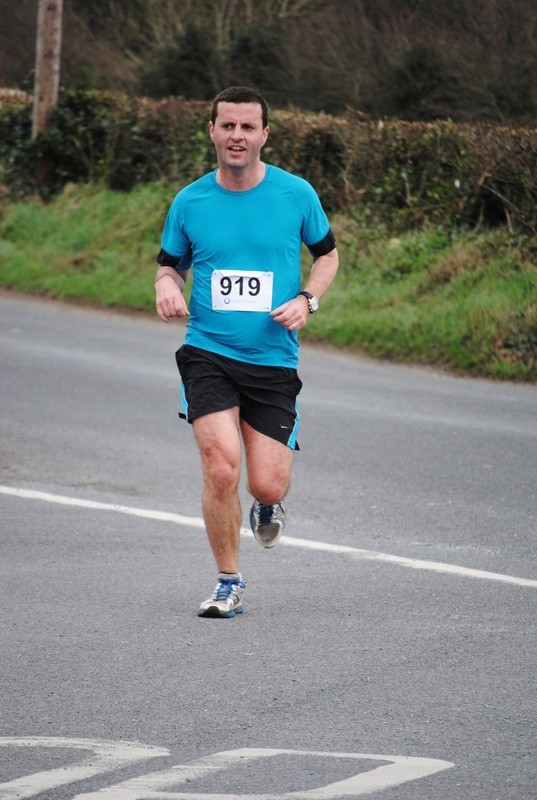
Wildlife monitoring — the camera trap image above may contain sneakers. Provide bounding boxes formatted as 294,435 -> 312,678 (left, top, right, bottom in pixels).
250,498 -> 287,549
197,571 -> 246,617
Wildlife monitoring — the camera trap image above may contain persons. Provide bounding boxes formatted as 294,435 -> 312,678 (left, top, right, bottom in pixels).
155,87 -> 339,618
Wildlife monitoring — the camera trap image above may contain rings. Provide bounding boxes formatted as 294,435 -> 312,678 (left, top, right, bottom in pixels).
162,307 -> 167,311
292,324 -> 296,329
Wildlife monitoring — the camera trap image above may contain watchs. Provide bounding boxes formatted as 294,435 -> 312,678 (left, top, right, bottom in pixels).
299,291 -> 319,314
